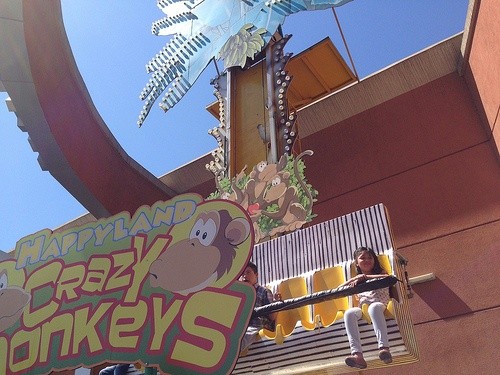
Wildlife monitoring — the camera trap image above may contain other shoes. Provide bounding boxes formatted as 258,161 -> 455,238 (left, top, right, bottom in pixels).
345,352 -> 368,370
379,348 -> 393,362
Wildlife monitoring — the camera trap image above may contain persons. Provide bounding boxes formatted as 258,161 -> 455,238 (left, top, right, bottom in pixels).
239,262 -> 277,358
337,247 -> 392,369
98,363 -> 160,375
